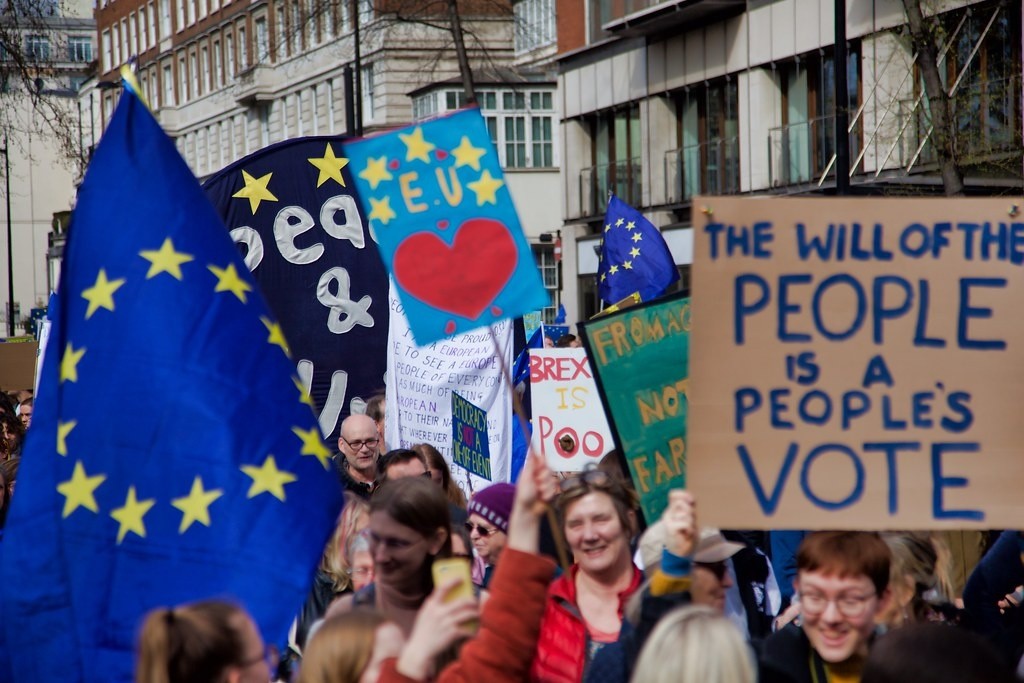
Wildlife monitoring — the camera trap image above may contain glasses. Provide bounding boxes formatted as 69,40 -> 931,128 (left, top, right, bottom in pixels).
558,470 -> 615,492
697,562 -> 728,580
342,437 -> 379,451
345,568 -> 370,581
423,471 -> 432,478
361,532 -> 424,552
796,585 -> 876,616
241,645 -> 280,668
464,520 -> 498,537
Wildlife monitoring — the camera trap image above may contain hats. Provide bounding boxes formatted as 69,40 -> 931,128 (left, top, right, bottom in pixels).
633,528 -> 746,576
467,483 -> 515,532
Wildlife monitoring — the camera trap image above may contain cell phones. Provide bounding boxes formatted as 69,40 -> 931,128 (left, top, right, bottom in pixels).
432,559 -> 478,635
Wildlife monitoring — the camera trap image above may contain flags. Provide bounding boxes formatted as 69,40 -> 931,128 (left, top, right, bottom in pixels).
596,196 -> 681,308
0,88 -> 340,683
512,326 -> 570,388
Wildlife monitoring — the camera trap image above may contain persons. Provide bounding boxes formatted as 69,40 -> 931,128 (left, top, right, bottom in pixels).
0,333 -> 1024,683
